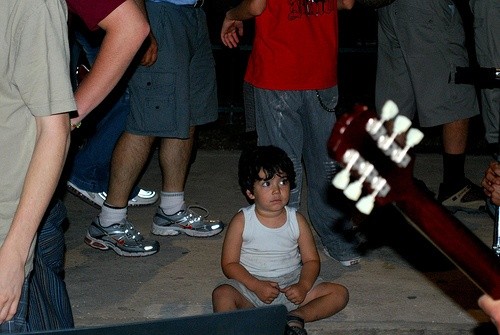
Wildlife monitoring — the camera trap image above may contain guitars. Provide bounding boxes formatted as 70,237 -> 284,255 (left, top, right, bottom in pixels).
326,99 -> 500,299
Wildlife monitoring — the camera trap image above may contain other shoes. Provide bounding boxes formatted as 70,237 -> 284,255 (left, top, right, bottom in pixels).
58,179 -> 107,209
128,189 -> 160,207
437,179 -> 487,213
284,316 -> 308,335
323,245 -> 360,266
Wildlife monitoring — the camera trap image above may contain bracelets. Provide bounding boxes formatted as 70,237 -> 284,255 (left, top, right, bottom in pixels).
70,121 -> 81,129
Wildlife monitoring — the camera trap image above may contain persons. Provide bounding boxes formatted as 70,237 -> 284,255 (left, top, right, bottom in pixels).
375,0 -> 500,335
0,0 -> 223,333
220,0 -> 361,266
212,145 -> 349,335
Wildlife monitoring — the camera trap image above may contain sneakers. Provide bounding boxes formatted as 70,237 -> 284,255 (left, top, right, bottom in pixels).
151,205 -> 224,238
84,215 -> 160,257
